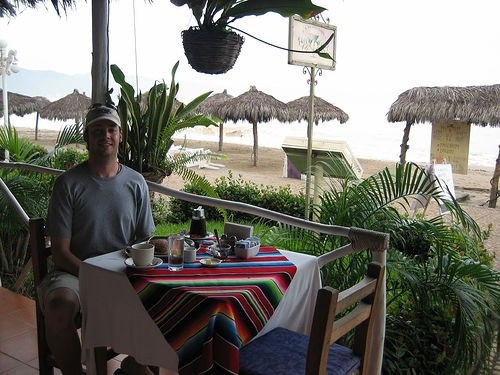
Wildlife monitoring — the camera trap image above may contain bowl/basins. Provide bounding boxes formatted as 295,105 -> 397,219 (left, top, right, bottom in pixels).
212,242 -> 231,259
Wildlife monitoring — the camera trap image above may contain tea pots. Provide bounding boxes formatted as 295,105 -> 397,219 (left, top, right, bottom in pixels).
189,206 -> 207,238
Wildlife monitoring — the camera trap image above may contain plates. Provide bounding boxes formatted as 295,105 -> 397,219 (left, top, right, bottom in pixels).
184,232 -> 214,240
125,240 -> 168,258
124,257 -> 164,268
200,258 -> 221,266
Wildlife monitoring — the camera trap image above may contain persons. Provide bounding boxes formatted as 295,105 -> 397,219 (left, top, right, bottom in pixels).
35,104 -> 157,375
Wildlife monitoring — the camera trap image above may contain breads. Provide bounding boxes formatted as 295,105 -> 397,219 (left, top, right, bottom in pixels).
150,239 -> 168,256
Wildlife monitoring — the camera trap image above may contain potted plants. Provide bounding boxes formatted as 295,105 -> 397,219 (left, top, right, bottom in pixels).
169,0 -> 327,75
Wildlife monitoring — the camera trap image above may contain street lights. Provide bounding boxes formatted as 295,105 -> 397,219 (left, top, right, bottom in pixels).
0,40 -> 20,164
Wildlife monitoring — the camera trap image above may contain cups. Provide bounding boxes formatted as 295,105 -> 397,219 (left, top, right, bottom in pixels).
125,243 -> 155,266
184,247 -> 197,263
167,236 -> 184,271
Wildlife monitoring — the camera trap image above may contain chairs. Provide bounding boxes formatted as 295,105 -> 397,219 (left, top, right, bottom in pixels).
30,217 -> 122,374
239,261 -> 386,375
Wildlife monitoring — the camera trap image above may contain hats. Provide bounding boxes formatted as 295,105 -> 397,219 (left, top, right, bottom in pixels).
86,104 -> 121,128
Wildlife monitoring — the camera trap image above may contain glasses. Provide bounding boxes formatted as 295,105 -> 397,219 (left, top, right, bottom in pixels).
86,102 -> 119,116
